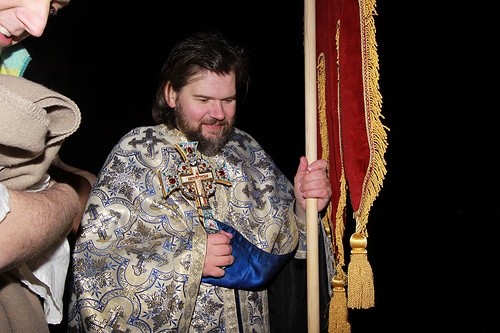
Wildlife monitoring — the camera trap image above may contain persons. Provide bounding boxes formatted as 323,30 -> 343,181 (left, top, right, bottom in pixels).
67,35 -> 332,331
2,1 -> 99,332
